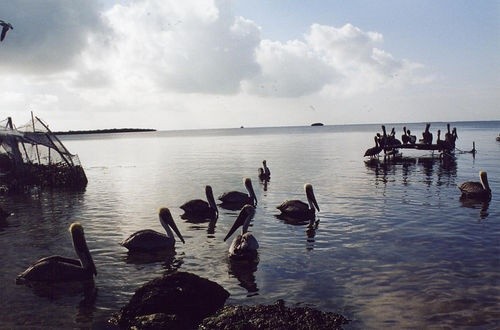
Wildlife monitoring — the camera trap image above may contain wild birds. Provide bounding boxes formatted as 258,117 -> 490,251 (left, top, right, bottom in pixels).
445,123 -> 452,144
258,160 -> 270,179
459,172 -> 492,194
217,178 -> 258,207
407,130 -> 416,144
436,129 -> 446,143
224,204 -> 259,257
16,223 -> 98,290
452,127 -> 458,143
402,126 -> 409,144
118,208 -> 185,250
277,184 -> 321,217
419,123 -> 433,144
364,125 -> 402,157
181,185 -> 219,216
